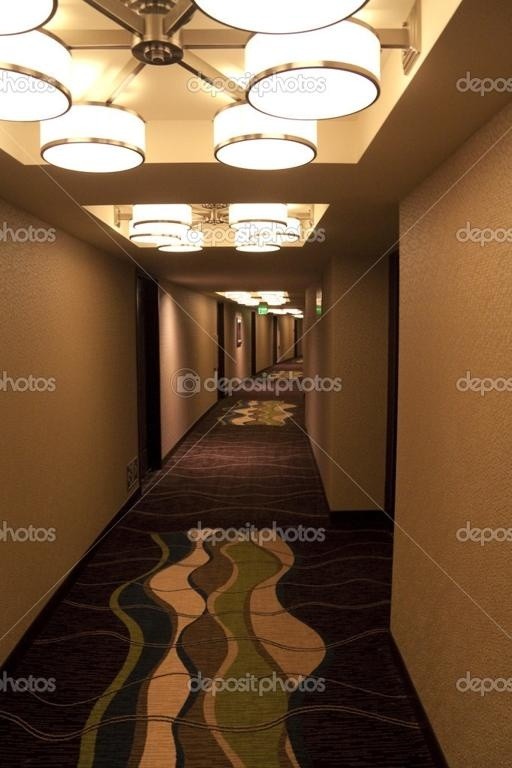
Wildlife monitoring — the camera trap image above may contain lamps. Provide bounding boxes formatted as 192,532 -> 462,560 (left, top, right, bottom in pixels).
0,0 -> 428,176
113,201 -> 314,255
